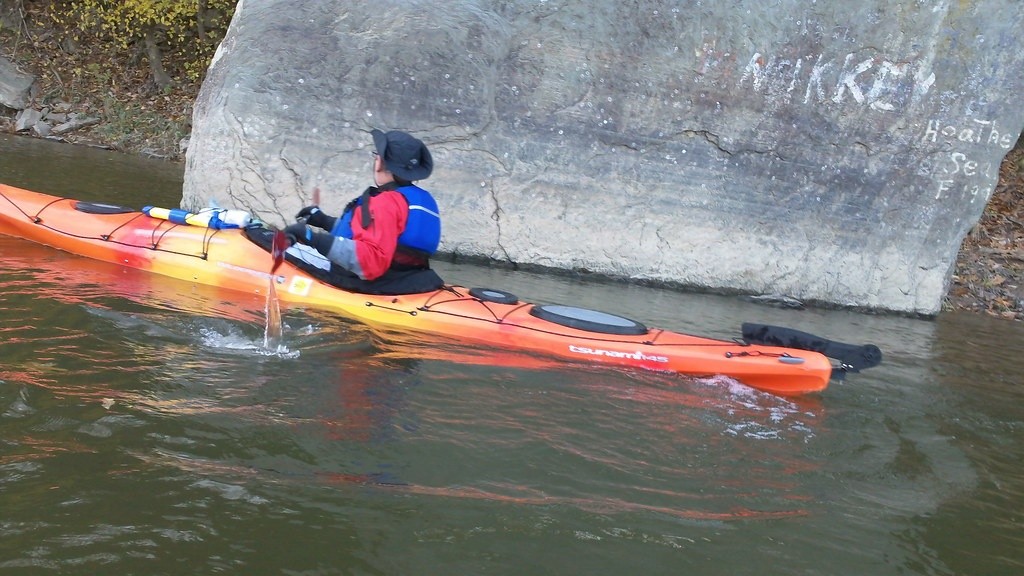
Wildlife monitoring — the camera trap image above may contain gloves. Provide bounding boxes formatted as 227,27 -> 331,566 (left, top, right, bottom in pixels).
282,222 -> 334,256
296,205 -> 337,228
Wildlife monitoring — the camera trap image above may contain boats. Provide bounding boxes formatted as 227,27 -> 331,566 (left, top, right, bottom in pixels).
0,182 -> 832,394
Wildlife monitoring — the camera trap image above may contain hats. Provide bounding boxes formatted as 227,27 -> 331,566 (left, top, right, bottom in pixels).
372,129 -> 433,181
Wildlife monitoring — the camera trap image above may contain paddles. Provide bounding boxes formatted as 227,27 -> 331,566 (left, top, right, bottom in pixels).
268,207 -> 314,272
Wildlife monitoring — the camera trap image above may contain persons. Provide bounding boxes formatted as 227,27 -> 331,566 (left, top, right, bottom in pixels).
286,130 -> 442,280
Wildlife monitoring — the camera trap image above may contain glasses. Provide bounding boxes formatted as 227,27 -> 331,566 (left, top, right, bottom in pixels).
371,150 -> 384,159
